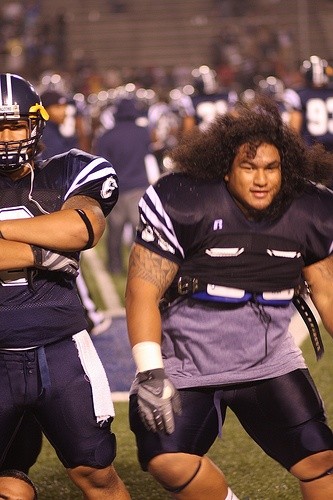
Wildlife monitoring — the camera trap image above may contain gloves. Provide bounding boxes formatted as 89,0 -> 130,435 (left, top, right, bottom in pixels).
25,244 -> 80,292
136,367 -> 183,435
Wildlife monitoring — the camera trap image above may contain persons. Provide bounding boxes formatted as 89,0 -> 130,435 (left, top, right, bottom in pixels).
40,55 -> 333,280
0,71 -> 131,499
124,100 -> 332,500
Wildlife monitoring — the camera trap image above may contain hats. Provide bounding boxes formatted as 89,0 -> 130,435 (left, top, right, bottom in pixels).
113,99 -> 141,118
41,92 -> 76,107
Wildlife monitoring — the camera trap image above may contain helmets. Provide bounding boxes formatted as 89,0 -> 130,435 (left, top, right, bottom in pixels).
0,73 -> 49,173
